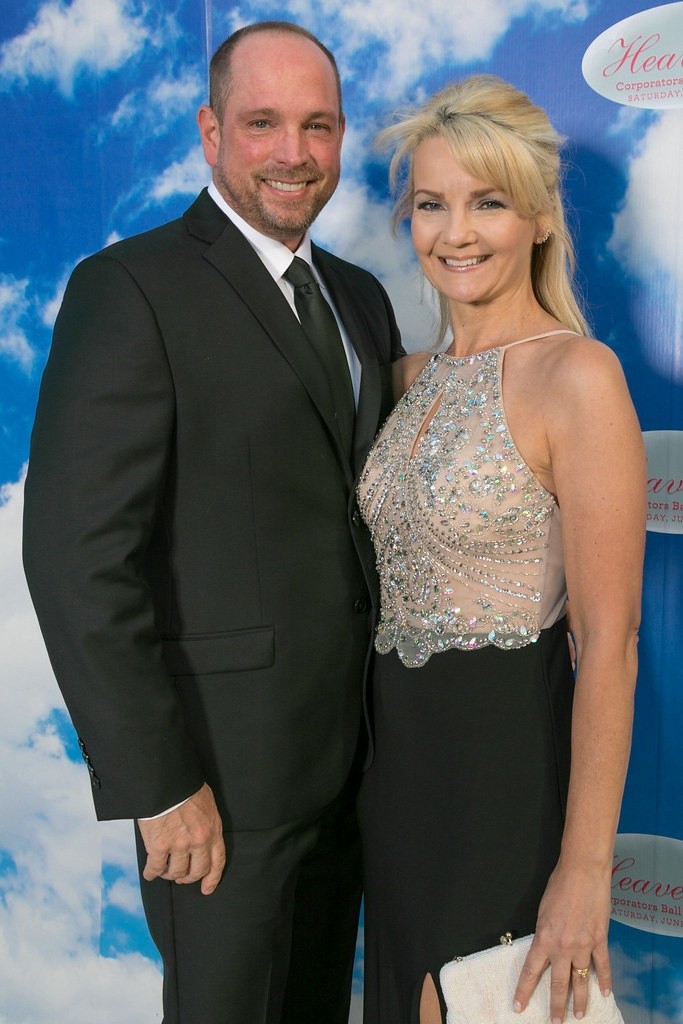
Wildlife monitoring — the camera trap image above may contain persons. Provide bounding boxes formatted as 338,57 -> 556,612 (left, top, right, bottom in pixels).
355,75 -> 647,1024
23,21 -> 576,1024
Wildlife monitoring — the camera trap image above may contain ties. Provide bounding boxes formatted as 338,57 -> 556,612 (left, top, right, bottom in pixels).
280,255 -> 353,468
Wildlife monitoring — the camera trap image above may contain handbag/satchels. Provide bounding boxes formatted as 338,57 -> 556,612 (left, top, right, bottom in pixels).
440,932 -> 630,1023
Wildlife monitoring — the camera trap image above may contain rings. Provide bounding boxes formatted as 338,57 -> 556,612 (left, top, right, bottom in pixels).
572,966 -> 589,978
